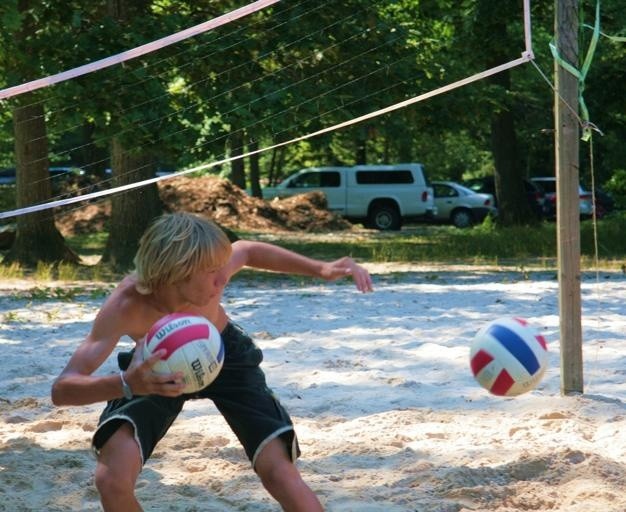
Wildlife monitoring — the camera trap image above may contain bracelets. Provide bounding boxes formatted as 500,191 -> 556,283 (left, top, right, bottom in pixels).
119,371 -> 133,403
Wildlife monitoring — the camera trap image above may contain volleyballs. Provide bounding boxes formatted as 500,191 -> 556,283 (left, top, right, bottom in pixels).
468,317 -> 549,398
143,313 -> 225,394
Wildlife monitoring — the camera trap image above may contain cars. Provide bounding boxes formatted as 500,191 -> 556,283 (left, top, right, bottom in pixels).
0,166 -> 98,188
429,176 -> 595,228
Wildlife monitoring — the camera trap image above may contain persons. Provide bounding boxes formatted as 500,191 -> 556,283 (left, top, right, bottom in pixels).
47,207 -> 377,510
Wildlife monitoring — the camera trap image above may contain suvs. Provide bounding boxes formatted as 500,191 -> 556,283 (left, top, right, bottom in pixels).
241,162 -> 435,230
